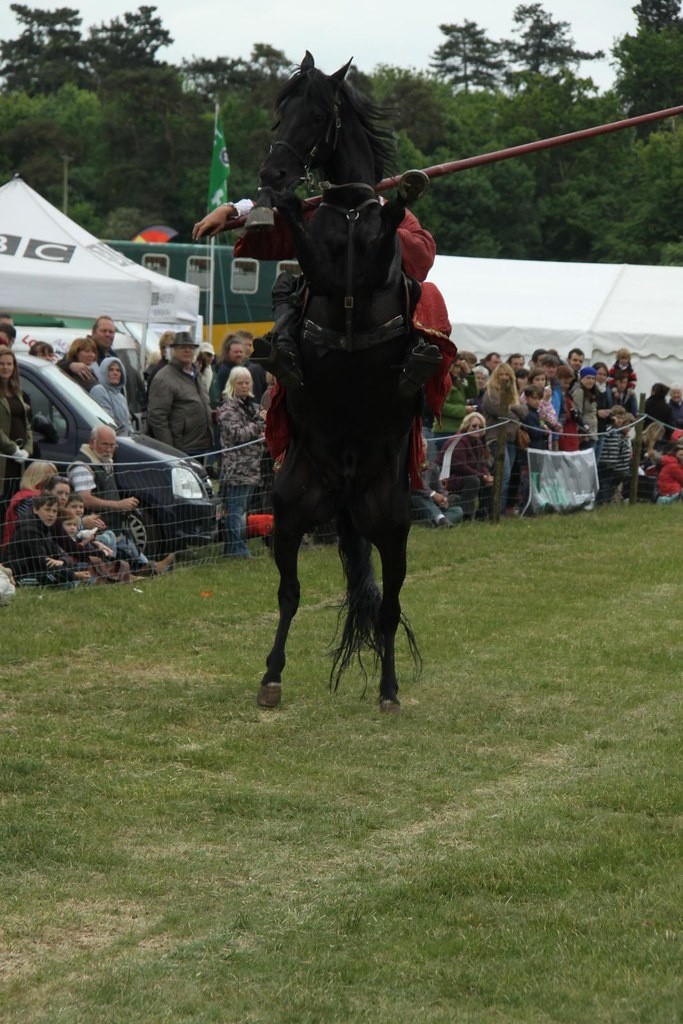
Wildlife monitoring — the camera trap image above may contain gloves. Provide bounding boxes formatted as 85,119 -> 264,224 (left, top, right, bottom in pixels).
12,446 -> 29,462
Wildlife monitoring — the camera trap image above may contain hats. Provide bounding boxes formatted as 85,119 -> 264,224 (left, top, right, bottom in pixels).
199,342 -> 215,356
169,331 -> 199,347
580,367 -> 596,379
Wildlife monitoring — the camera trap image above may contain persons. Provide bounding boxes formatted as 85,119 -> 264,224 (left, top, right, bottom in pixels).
192,157 -> 459,491
0,302 -> 283,588
413,322 -> 683,534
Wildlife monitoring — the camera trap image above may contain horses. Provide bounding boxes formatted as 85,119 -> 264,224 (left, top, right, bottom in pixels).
243,51 -> 433,713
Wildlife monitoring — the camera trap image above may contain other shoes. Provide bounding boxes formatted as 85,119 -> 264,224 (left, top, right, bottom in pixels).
439,517 -> 454,528
143,553 -> 175,576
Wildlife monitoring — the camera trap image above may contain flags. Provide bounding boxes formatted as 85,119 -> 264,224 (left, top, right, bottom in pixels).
206,107 -> 231,215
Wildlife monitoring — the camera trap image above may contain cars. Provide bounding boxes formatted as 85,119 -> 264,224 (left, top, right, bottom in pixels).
8,351 -> 220,563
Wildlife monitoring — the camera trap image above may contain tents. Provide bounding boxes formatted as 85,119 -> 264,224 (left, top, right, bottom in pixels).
422,249 -> 683,389
0,172 -> 202,376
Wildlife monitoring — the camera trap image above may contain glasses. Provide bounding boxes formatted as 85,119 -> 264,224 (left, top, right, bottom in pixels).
472,425 -> 483,429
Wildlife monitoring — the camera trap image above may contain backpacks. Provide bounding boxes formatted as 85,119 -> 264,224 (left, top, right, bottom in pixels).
85,556 -> 130,586
